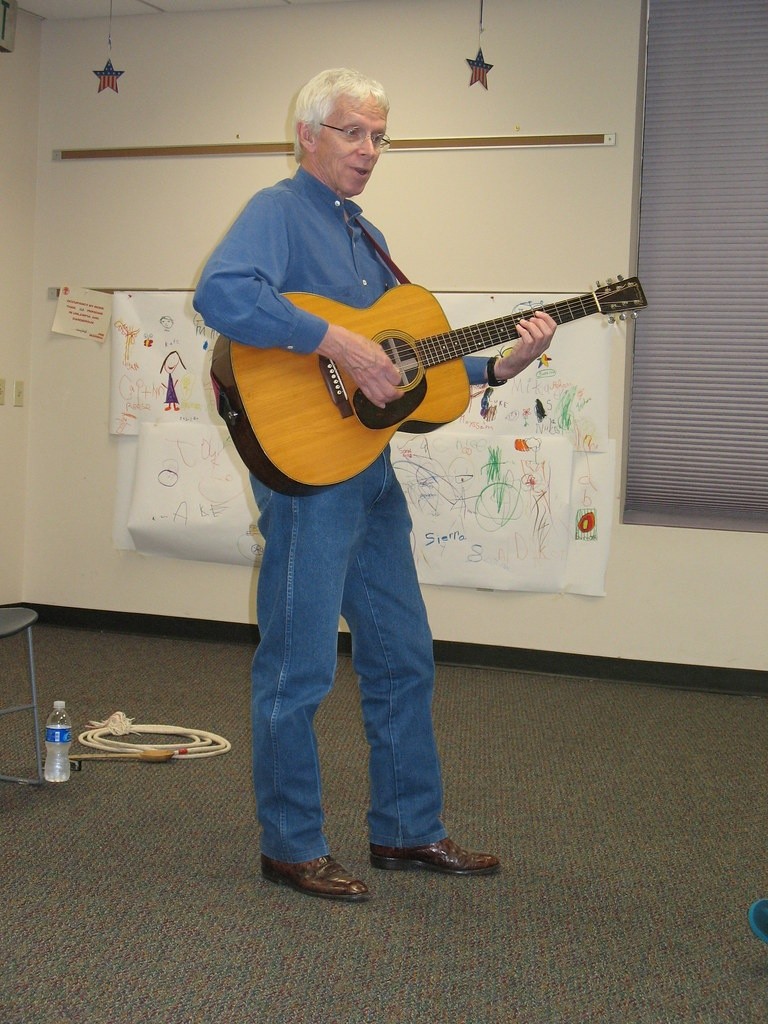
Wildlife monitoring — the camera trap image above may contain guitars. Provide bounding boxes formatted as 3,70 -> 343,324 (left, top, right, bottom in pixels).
211,273 -> 650,499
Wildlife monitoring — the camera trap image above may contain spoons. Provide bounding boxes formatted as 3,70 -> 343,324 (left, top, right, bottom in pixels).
69,749 -> 174,763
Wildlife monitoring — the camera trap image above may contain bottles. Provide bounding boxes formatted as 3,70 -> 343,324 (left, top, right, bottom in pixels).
44,700 -> 72,783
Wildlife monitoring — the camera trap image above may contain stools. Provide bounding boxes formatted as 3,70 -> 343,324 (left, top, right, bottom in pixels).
0,607 -> 45,786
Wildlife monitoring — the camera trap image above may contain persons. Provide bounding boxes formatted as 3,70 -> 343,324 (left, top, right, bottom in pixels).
193,66 -> 558,902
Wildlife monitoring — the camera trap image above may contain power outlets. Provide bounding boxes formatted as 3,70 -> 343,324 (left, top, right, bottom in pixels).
0,378 -> 6,406
13,380 -> 25,407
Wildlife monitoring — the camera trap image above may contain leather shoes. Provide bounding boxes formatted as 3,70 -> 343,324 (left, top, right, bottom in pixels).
368,836 -> 500,875
261,850 -> 369,900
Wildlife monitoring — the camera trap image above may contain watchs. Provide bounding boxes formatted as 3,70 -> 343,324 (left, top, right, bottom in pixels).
487,355 -> 508,387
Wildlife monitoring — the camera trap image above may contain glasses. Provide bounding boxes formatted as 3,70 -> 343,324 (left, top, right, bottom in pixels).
319,122 -> 391,154
